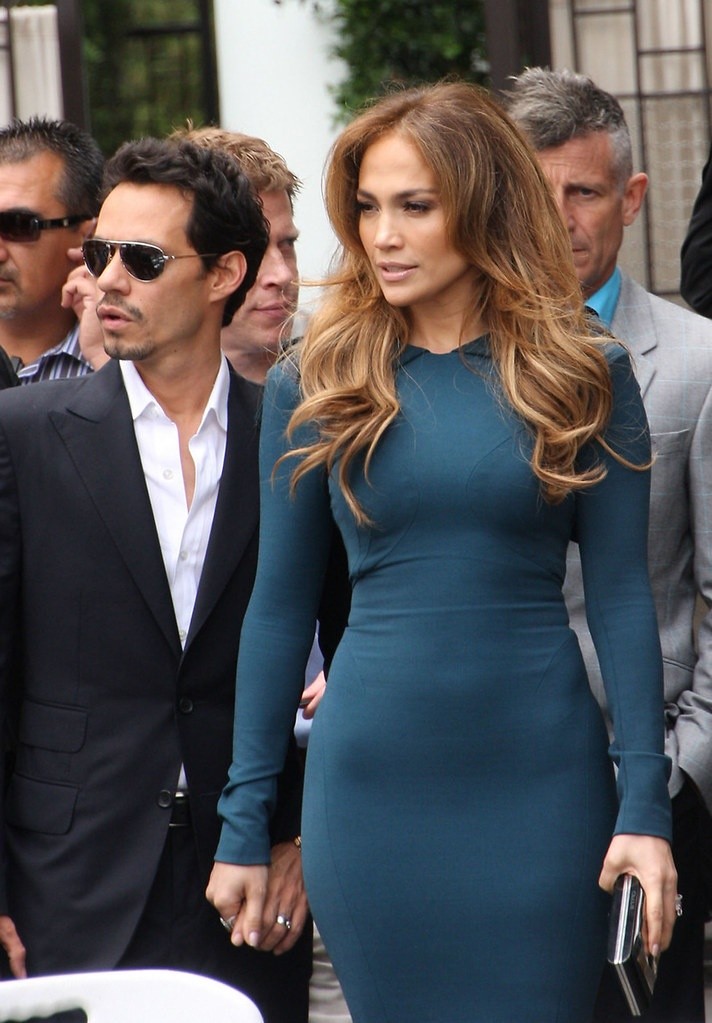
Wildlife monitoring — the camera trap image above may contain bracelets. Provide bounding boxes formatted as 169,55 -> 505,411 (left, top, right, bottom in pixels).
294,836 -> 301,851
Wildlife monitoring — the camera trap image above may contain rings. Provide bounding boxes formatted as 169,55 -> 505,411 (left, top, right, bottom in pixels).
276,915 -> 291,929
219,916 -> 236,932
674,894 -> 683,916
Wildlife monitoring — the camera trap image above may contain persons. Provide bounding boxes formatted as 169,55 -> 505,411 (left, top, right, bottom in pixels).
0,132 -> 349,1022
504,67 -> 712,1023
677,145 -> 711,319
1,117 -> 113,388
204,86 -> 678,1023
166,120 -> 324,750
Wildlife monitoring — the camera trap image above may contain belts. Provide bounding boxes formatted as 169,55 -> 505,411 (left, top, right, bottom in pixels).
167,792 -> 187,827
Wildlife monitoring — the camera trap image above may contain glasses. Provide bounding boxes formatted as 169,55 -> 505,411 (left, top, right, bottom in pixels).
81,238 -> 230,282
0,211 -> 100,243
